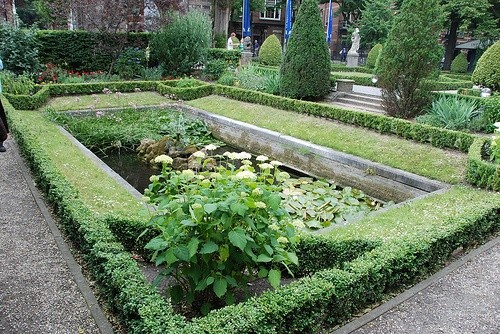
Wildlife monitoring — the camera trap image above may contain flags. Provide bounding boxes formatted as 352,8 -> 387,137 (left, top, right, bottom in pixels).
244,0 -> 251,37
327,0 -> 332,42
285,0 -> 291,38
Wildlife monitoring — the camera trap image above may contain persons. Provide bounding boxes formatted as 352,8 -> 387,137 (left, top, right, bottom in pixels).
340,47 -> 347,61
0,59 -> 10,152
254,40 -> 259,56
349,28 -> 361,52
231,33 -> 239,49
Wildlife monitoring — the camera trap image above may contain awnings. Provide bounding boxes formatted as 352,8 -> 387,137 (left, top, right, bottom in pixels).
455,39 -> 492,50
